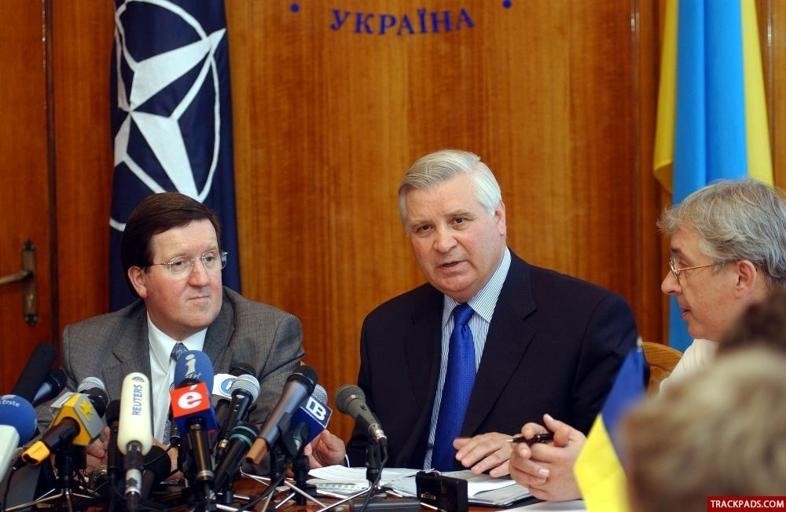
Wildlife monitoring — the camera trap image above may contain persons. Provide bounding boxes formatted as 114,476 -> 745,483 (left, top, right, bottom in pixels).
509,178 -> 786,512
34,191 -> 304,477
295,149 -> 649,480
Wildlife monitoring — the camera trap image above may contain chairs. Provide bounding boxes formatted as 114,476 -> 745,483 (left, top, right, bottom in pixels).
643,342 -> 683,393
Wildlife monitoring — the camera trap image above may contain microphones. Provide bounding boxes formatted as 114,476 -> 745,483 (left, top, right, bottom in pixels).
76,377 -> 105,392
168,377 -> 218,512
217,373 -> 261,450
0,394 -> 38,483
31,367 -> 69,408
283,382 -> 333,502
245,364 -> 318,466
205,423 -> 259,501
141,444 -> 172,509
104,399 -> 130,500
334,383 -> 387,447
116,372 -> 153,512
22,387 -> 109,466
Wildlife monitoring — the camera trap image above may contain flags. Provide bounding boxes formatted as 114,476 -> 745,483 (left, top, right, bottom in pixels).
574,344 -> 652,512
109,2 -> 243,312
652,0 -> 776,353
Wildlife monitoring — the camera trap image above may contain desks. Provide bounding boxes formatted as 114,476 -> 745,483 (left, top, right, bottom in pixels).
44,475 -> 506,512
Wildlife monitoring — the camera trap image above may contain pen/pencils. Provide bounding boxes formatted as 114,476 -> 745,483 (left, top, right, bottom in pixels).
506,433 -> 554,443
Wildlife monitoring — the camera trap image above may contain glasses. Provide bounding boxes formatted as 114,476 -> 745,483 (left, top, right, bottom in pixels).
668,256 -> 738,279
146,251 -> 227,277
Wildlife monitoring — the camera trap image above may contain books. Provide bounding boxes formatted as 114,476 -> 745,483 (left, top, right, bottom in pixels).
308,455 -> 535,506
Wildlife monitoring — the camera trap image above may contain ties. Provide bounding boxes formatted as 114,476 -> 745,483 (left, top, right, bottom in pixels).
164,343 -> 189,446
431,305 -> 477,472
